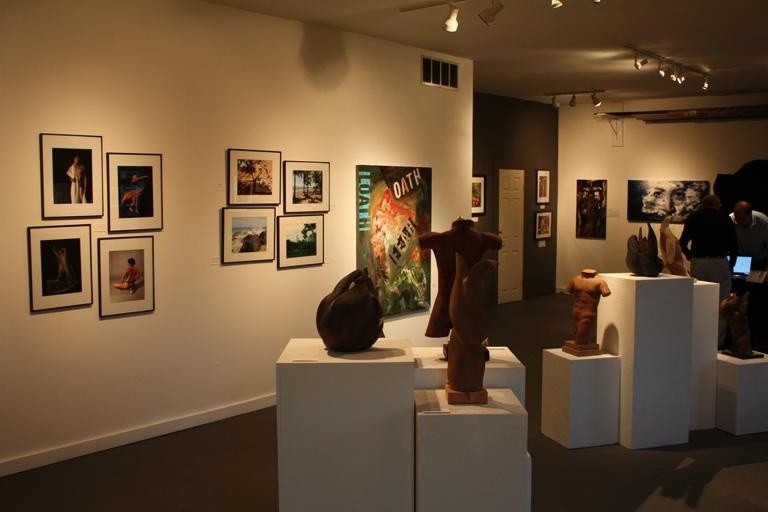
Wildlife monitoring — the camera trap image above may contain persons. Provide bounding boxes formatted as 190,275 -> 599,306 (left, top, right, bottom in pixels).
728,201 -> 768,273
679,195 -> 737,347
419,219 -> 503,336
121,174 -> 149,213
113,258 -> 141,294
52,243 -> 72,285
66,154 -> 89,204
566,268 -> 611,346
640,181 -> 686,217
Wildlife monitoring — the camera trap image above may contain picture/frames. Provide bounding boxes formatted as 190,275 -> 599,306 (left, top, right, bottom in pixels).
471,176 -> 487,217
26,224 -> 93,316
106,153 -> 164,234
535,211 -> 552,240
536,169 -> 551,204
227,148 -> 282,206
220,207 -> 276,266
97,235 -> 156,320
283,160 -> 330,214
276,215 -> 324,271
40,132 -> 104,220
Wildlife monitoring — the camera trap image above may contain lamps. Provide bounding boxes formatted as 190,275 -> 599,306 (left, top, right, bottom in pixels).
552,89 -> 601,109
549,0 -> 563,9
440,1 -> 459,33
477,0 -> 505,29
633,42 -> 712,95
592,0 -> 601,7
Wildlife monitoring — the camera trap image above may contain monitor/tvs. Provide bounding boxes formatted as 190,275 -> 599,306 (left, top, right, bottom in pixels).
726,254 -> 753,276
